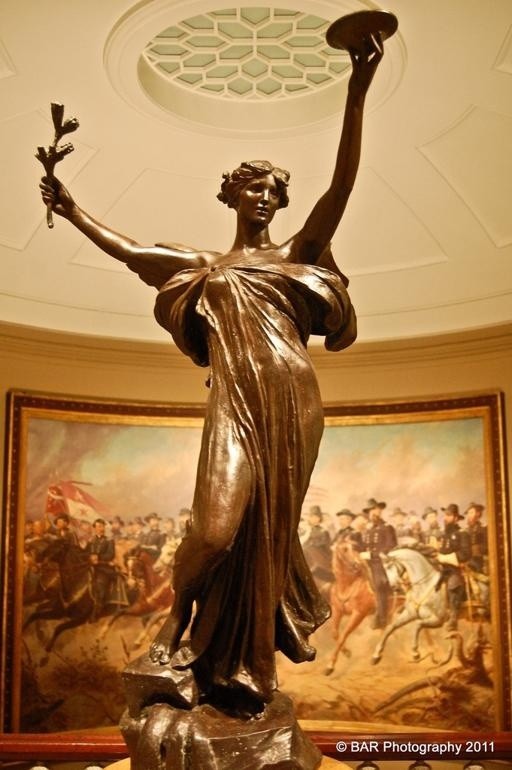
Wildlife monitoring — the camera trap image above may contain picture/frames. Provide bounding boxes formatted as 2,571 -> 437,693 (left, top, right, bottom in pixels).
0,386 -> 512,762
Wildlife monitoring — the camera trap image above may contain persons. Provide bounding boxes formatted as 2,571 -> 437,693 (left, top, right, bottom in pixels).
27,30 -> 406,725
25,496 -> 488,632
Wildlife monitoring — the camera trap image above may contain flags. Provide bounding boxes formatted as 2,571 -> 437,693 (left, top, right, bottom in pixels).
62,477 -> 111,526
44,484 -> 71,516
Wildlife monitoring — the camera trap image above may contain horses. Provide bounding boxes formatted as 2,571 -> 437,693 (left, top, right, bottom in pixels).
23,534 -> 487,677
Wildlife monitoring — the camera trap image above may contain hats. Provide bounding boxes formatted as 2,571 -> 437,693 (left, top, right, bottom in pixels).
300,498 -> 485,521
23,508 -> 193,527
